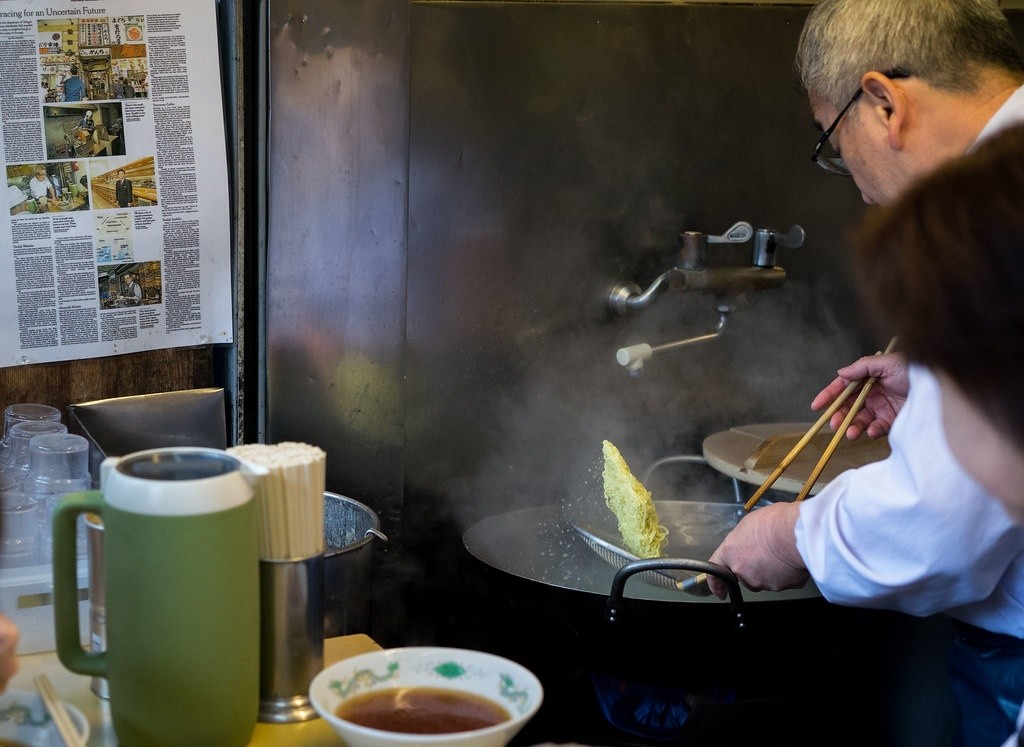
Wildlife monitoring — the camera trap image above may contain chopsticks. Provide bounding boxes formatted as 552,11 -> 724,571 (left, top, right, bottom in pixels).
34,673 -> 84,747
228,442 -> 326,563
743,334 -> 899,513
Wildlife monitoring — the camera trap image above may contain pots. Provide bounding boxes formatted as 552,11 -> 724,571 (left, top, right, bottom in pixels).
459,454 -> 889,679
325,490 -> 388,640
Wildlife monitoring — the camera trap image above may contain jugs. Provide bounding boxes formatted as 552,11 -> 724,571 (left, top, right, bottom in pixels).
51,447 -> 270,747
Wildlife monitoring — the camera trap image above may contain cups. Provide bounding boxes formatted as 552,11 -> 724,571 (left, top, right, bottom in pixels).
259,550 -> 329,723
0,404 -> 92,568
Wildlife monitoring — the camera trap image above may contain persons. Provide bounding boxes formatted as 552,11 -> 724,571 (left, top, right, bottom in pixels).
114,70 -> 149,99
117,273 -> 141,306
29,165 -> 56,202
850,119 -> 1024,747
60,65 -> 85,101
116,169 -> 133,208
72,111 -> 95,135
706,0 -> 1024,643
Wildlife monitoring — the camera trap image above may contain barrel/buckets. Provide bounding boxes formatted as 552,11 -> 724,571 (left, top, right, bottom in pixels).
84,511 -> 111,699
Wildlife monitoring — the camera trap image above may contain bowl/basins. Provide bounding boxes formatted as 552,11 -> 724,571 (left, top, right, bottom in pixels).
308,647 -> 544,747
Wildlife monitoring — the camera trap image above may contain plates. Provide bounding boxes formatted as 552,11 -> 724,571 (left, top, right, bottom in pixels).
0,689 -> 90,747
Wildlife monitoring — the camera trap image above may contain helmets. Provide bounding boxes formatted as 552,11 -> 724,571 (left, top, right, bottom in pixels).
86,110 -> 93,115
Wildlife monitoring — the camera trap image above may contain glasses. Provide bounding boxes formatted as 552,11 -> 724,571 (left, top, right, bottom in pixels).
38,173 -> 47,176
811,66 -> 910,175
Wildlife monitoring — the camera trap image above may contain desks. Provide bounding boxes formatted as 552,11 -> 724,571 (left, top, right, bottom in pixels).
7,634 -> 385,747
